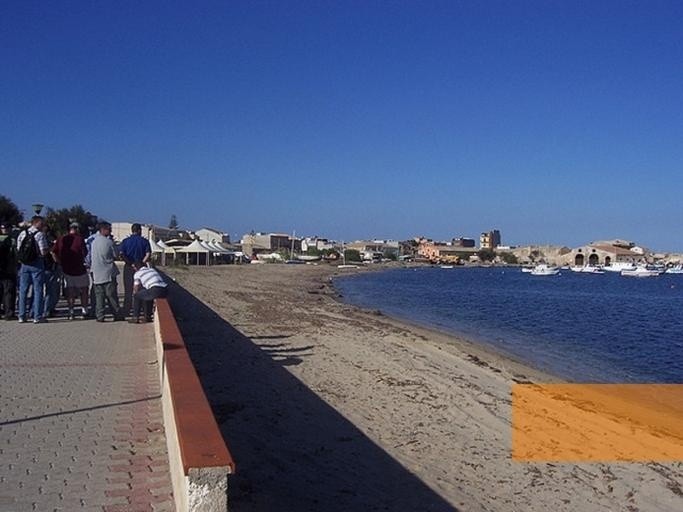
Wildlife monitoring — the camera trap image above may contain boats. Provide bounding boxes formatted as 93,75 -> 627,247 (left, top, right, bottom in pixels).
521,265 -> 673,278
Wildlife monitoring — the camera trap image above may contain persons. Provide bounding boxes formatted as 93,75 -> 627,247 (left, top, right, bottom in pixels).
0,214 -> 167,324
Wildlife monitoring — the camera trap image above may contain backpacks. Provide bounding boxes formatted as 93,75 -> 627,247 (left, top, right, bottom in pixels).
18,228 -> 41,267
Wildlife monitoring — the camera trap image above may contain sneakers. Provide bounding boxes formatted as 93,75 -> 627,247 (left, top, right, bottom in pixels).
68,312 -> 92,320
4,313 -> 49,323
112,315 -> 151,323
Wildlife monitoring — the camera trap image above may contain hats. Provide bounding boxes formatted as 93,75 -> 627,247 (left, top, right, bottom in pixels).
70,222 -> 83,228
1,221 -> 14,229
